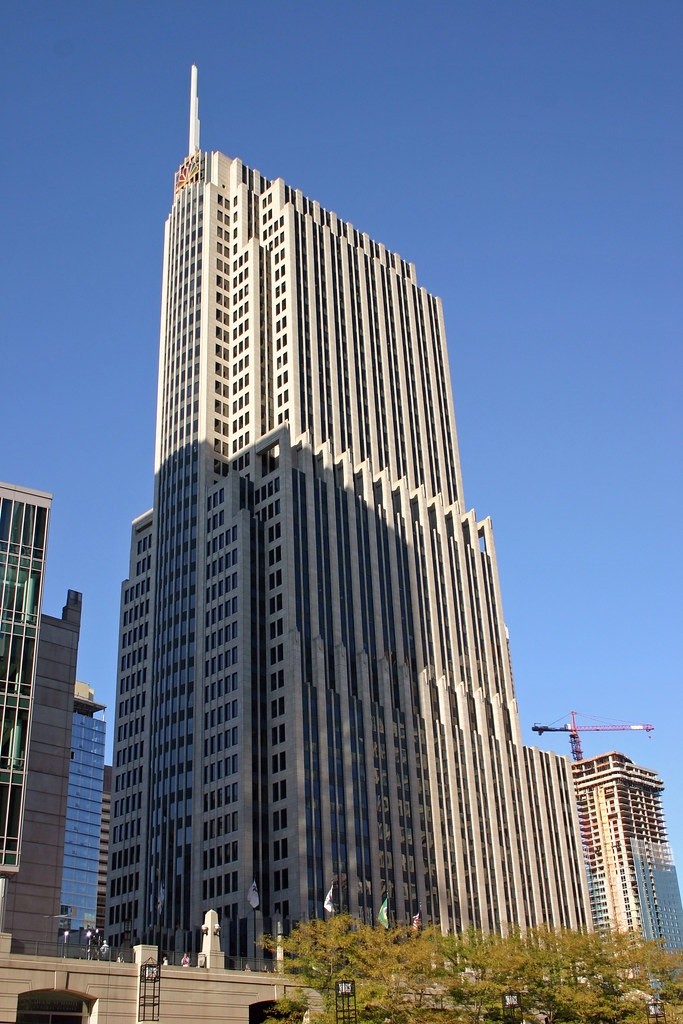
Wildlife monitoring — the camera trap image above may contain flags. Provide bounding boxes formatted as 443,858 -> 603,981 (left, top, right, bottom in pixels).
413,910 -> 422,929
247,880 -> 260,908
324,885 -> 336,912
378,899 -> 389,929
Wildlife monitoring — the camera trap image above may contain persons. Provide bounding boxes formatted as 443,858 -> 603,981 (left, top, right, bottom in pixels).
88,935 -> 109,961
243,963 -> 271,972
162,956 -> 170,965
180,953 -> 190,966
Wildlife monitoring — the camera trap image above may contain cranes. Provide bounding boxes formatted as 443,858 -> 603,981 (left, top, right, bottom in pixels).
532,710 -> 656,763
